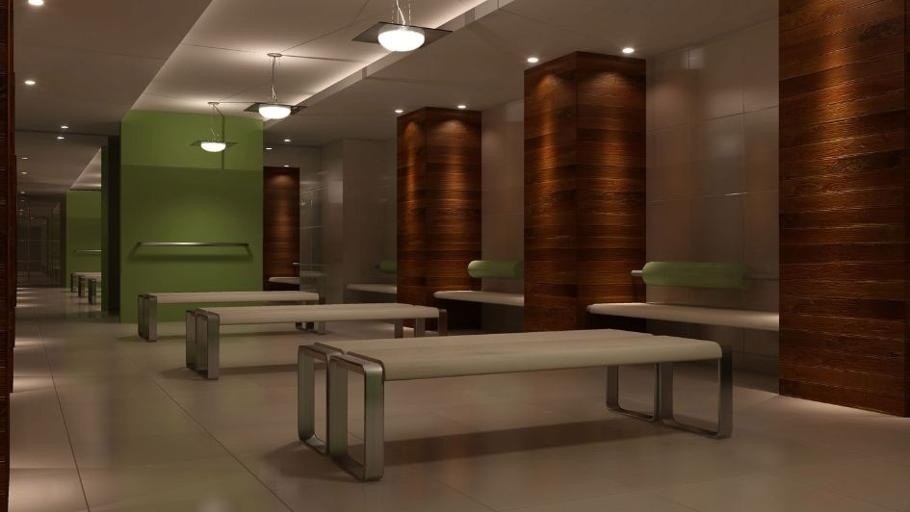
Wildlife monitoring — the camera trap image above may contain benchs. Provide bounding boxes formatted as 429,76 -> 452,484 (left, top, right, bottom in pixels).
185,301 -> 446,379
296,330 -> 738,483
347,283 -> 397,294
586,302 -> 780,333
268,276 -> 300,284
70,271 -> 101,305
434,289 -> 525,310
136,292 -> 320,342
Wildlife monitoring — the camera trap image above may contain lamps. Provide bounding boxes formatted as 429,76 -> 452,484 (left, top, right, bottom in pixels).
242,52 -> 308,121
350,0 -> 452,54
191,102 -> 237,156
